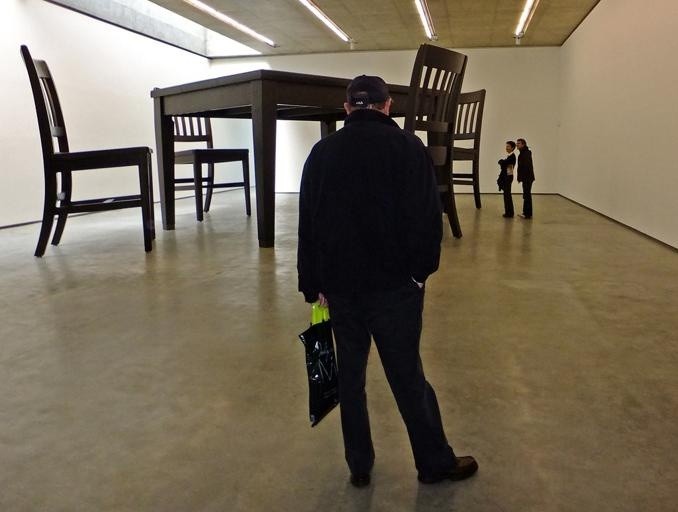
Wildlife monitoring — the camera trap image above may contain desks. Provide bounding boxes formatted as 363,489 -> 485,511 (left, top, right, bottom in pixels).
150,71 -> 452,245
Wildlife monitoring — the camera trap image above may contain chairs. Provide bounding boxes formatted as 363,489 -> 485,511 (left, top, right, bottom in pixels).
155,87 -> 251,220
18,45 -> 159,257
402,43 -> 488,240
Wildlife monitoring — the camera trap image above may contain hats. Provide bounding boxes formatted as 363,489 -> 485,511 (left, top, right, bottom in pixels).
346,74 -> 394,109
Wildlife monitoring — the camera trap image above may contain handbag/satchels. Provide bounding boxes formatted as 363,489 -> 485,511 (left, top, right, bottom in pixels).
296,300 -> 348,428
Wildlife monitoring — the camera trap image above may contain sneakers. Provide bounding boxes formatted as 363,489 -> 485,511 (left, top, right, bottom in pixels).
350,471 -> 373,490
503,213 -> 531,218
417,454 -> 480,485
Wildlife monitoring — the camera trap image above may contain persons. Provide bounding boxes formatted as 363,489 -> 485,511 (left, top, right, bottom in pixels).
297,74 -> 477,488
497,141 -> 517,218
516,138 -> 535,219
